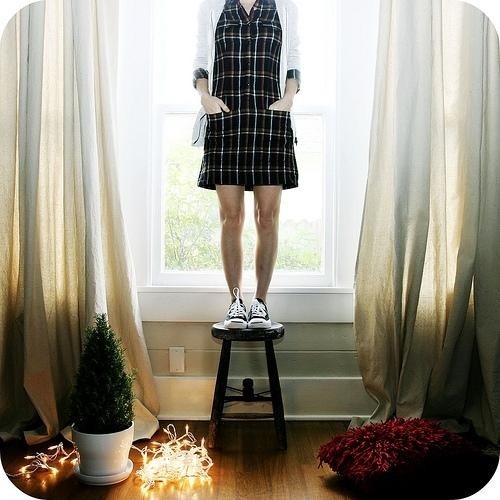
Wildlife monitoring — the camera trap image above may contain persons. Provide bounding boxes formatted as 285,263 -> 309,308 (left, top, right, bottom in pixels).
190,0 -> 302,333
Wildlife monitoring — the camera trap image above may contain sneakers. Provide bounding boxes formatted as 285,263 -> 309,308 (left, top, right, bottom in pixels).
247,298 -> 273,329
224,287 -> 248,329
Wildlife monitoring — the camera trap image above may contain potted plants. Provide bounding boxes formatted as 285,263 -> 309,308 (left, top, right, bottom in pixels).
69,310 -> 139,485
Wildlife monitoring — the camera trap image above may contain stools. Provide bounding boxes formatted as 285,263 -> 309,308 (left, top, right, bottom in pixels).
205,319 -> 293,455
